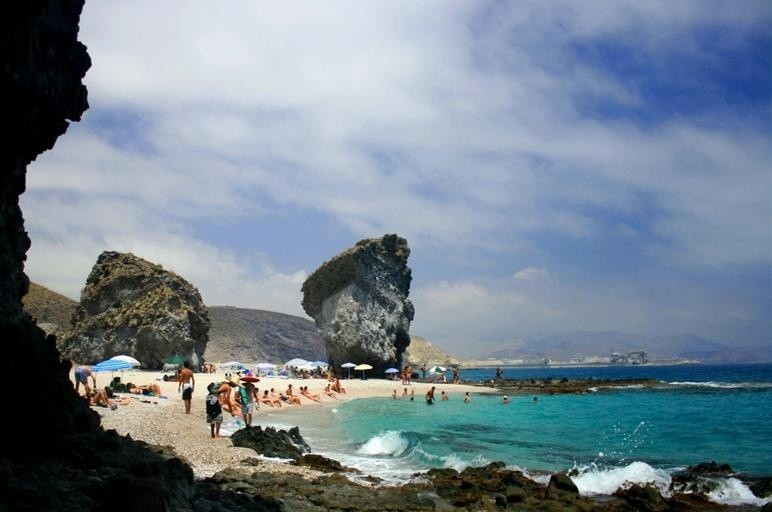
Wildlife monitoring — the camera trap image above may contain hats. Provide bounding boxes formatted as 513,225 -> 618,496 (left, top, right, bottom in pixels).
240,373 -> 260,382
226,375 -> 246,389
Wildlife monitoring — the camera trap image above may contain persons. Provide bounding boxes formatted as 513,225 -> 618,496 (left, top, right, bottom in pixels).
452,368 -> 458,384
406,366 -> 412,384
422,363 -> 427,378
385,373 -> 394,380
75,355 -> 347,438
425,386 -> 435,405
502,395 -> 509,404
437,371 -> 444,383
401,366 -> 408,385
394,373 -> 401,380
409,389 -> 415,400
441,390 -> 448,401
463,392 -> 471,403
393,388 -> 398,399
500,371 -> 504,379
532,395 -> 538,403
496,367 -> 501,379
402,388 -> 408,398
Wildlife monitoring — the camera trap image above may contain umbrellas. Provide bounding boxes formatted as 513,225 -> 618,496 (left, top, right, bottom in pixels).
354,363 -> 374,380
429,365 -> 447,374
341,361 -> 356,381
384,366 -> 401,378
